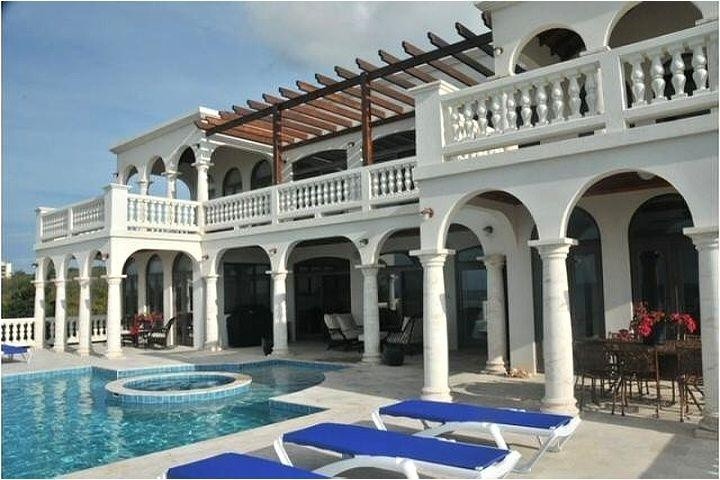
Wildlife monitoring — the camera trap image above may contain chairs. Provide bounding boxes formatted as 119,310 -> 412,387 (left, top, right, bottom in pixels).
274,422 -> 522,479
167,453 -> 328,479
121,317 -> 176,348
371,399 -> 583,475
574,334 -> 702,422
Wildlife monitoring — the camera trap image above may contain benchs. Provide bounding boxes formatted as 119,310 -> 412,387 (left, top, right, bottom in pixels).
1,345 -> 30,363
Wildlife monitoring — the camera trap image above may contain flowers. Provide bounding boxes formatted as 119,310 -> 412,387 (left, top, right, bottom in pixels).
131,311 -> 164,335
629,306 -> 697,338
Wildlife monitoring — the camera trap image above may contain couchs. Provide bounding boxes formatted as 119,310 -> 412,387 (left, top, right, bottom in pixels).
321,312 -> 423,354
